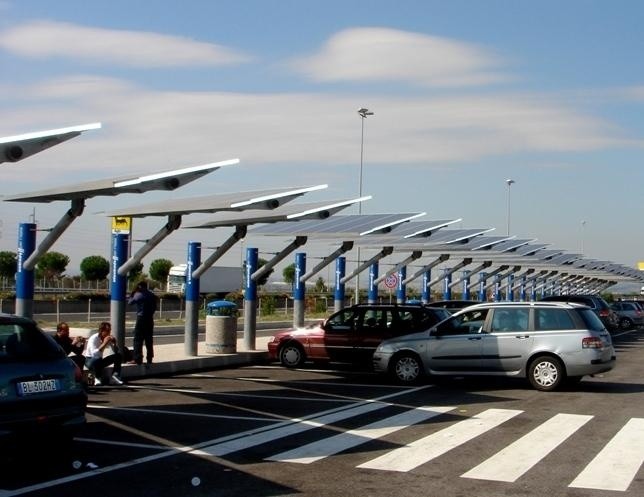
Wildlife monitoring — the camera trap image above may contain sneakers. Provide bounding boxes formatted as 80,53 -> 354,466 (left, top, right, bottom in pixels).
147,358 -> 152,364
128,360 -> 143,364
94,377 -> 102,386
110,375 -> 123,384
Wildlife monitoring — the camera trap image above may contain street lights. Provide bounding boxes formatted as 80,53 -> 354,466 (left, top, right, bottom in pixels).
354,107 -> 373,304
506,179 -> 515,301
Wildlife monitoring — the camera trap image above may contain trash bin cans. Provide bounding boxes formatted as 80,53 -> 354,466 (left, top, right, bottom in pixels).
205,301 -> 238,354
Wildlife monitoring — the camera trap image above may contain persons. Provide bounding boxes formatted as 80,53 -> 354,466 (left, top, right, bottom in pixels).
126,280 -> 159,366
51,323 -> 86,372
84,323 -> 124,385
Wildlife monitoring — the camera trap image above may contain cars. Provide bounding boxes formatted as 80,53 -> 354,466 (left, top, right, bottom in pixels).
0,312 -> 88,452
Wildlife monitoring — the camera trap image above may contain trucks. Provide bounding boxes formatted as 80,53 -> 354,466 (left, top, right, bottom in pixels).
165,264 -> 244,296
